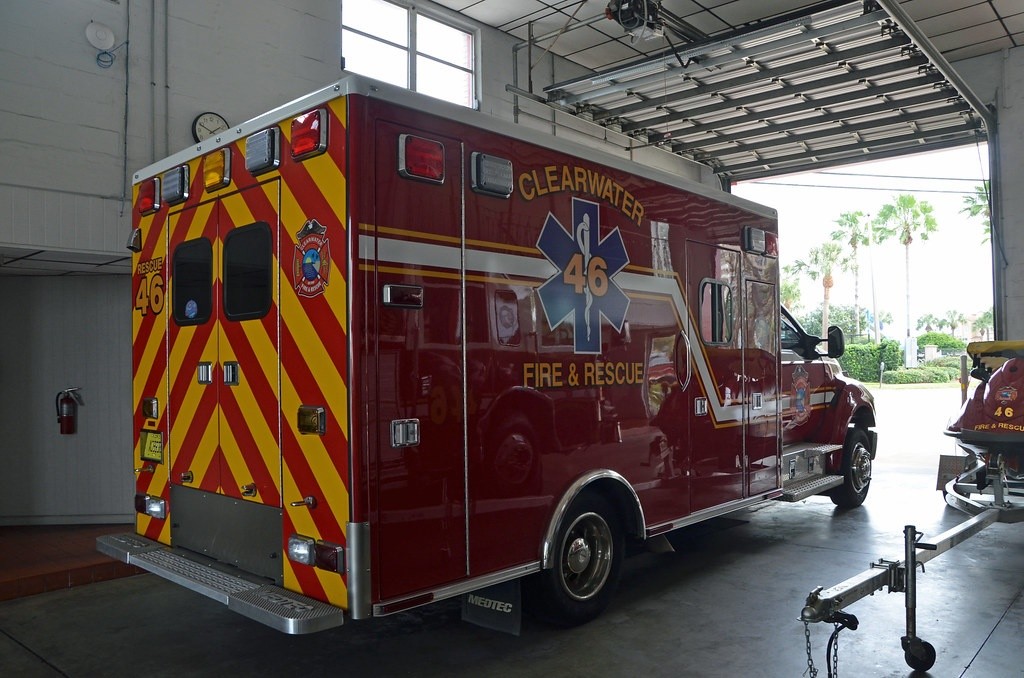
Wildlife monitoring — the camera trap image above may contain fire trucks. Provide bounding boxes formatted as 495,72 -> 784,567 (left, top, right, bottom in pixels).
92,73 -> 879,635
